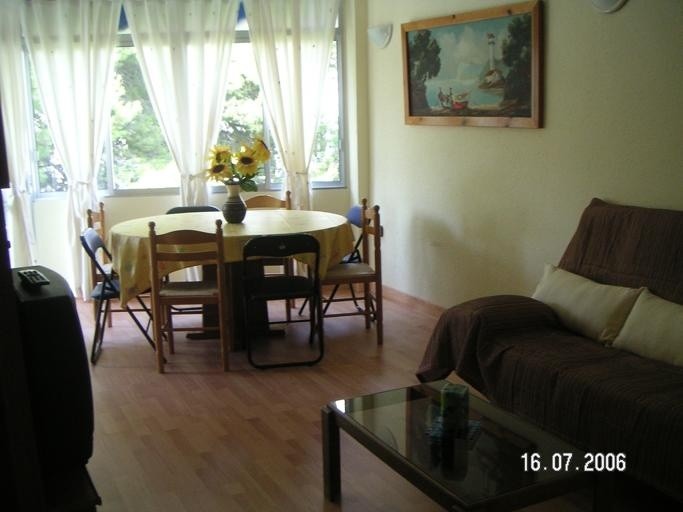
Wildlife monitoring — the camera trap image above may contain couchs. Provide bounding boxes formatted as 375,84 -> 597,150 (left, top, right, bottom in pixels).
414,198 -> 683,512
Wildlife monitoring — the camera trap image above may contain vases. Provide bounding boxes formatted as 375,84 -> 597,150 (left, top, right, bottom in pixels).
222,182 -> 247,223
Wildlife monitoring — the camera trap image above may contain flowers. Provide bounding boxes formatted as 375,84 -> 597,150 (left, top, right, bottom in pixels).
203,135 -> 271,192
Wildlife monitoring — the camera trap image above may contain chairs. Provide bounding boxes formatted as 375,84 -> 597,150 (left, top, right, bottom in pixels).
162,204 -> 221,333
305,197 -> 383,347
146,220 -> 237,372
86,201 -> 167,327
243,191 -> 296,321
297,204 -> 373,321
241,233 -> 325,369
80,227 -> 168,363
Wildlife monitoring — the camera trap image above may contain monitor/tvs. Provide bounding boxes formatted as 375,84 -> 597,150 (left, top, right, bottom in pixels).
12,265 -> 93,471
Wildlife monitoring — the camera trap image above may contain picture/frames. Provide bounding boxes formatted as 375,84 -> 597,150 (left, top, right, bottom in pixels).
401,1 -> 542,129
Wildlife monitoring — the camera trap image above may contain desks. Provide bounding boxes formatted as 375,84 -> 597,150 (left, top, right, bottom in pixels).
110,210 -> 356,340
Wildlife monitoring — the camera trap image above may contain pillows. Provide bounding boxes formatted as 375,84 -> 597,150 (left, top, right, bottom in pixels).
613,286 -> 683,368
531,263 -> 643,345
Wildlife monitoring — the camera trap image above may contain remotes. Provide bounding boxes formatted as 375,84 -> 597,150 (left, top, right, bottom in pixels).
18,270 -> 50,286
